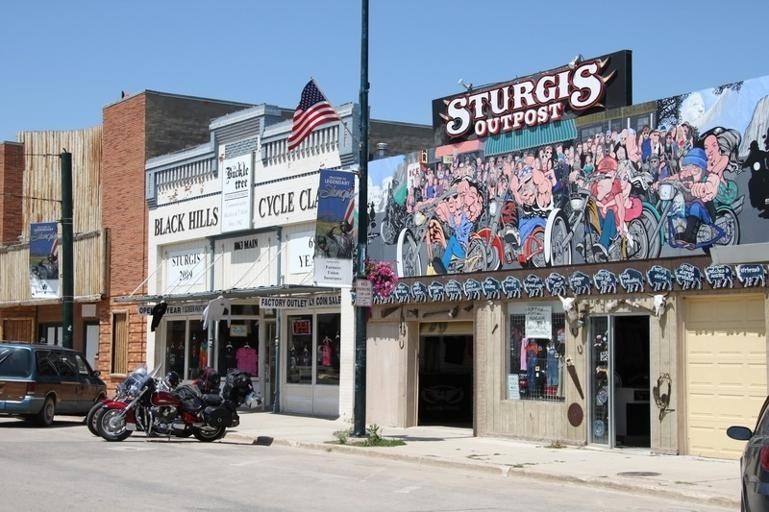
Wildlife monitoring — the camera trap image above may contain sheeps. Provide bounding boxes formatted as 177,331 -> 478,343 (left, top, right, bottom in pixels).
647,289 -> 670,319
555,289 -> 579,327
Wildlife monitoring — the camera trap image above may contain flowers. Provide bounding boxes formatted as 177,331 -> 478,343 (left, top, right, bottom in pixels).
359,255 -> 401,300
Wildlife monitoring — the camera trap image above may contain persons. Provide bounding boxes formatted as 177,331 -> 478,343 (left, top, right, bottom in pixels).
33,266 -> 38,275
327,221 -> 354,260
316,235 -> 329,257
39,253 -> 58,279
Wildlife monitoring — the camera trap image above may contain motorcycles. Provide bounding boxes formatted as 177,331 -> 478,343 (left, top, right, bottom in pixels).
96,370 -> 254,442
87,361 -> 222,438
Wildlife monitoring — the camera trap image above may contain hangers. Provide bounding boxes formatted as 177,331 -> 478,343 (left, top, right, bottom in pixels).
291,344 -> 297,351
304,344 -> 309,353
227,340 -> 232,350
322,334 -> 331,344
243,342 -> 249,348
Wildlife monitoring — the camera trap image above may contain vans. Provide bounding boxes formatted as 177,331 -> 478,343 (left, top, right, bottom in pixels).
1,340 -> 109,427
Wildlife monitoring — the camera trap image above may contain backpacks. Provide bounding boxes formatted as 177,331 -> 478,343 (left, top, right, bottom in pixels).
223,369 -> 253,404
197,366 -> 220,394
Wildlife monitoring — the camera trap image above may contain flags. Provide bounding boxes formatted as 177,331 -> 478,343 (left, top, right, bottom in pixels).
287,81 -> 339,151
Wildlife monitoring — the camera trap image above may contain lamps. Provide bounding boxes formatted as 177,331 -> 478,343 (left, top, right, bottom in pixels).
404,308 -> 420,320
447,304 -> 461,318
568,53 -> 585,73
456,76 -> 474,95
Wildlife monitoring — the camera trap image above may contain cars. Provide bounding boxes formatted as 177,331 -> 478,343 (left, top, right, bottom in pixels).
728,391 -> 768,512
